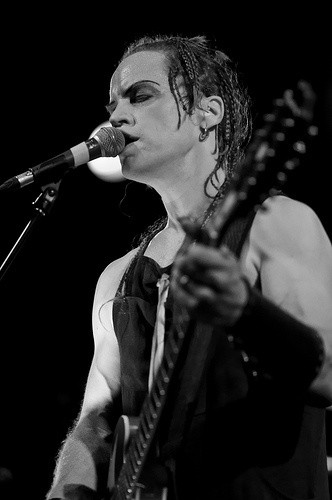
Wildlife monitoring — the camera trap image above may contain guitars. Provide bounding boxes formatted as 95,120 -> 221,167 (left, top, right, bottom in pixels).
106,80 -> 319,500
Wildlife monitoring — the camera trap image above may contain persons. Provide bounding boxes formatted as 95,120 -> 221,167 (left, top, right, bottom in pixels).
42,36 -> 332,500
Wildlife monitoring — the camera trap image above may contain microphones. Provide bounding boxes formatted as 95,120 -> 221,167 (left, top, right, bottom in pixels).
3,127 -> 125,193
